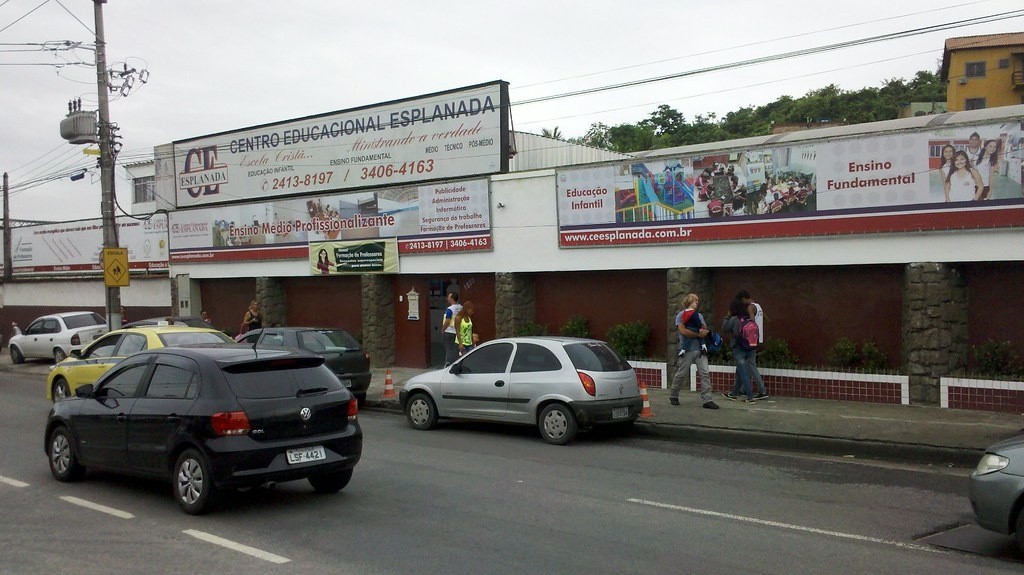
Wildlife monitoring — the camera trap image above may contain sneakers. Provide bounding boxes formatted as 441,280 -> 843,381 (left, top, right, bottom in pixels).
753,391 -> 769,399
736,391 -> 746,397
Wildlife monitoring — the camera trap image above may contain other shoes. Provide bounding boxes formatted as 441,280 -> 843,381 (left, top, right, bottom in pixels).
670,397 -> 679,405
702,344 -> 708,355
677,348 -> 686,357
741,398 -> 756,404
703,401 -> 720,409
721,391 -> 737,401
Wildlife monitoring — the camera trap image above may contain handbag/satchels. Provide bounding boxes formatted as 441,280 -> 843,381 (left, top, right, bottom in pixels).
234,323 -> 249,343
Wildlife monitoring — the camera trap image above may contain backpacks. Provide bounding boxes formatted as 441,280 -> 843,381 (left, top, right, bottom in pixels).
730,314 -> 760,350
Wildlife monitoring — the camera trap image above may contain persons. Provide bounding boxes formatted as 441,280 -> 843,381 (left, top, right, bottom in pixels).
722,292 -> 757,404
440,291 -> 480,365
12,321 -> 21,335
200,309 -> 213,324
243,300 -> 262,331
737,289 -> 769,400
940,132 -> 999,202
120,306 -> 129,325
694,161 -> 816,216
669,293 -> 719,409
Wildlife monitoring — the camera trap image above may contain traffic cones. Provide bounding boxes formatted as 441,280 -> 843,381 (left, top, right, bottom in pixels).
381,369 -> 397,398
638,382 -> 656,418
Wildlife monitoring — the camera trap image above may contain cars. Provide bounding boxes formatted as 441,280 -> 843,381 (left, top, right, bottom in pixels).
42,342 -> 364,514
967,434 -> 1024,550
7,311 -> 109,365
117,315 -> 232,338
397,334 -> 644,447
46,324 -> 236,407
236,325 -> 372,405
